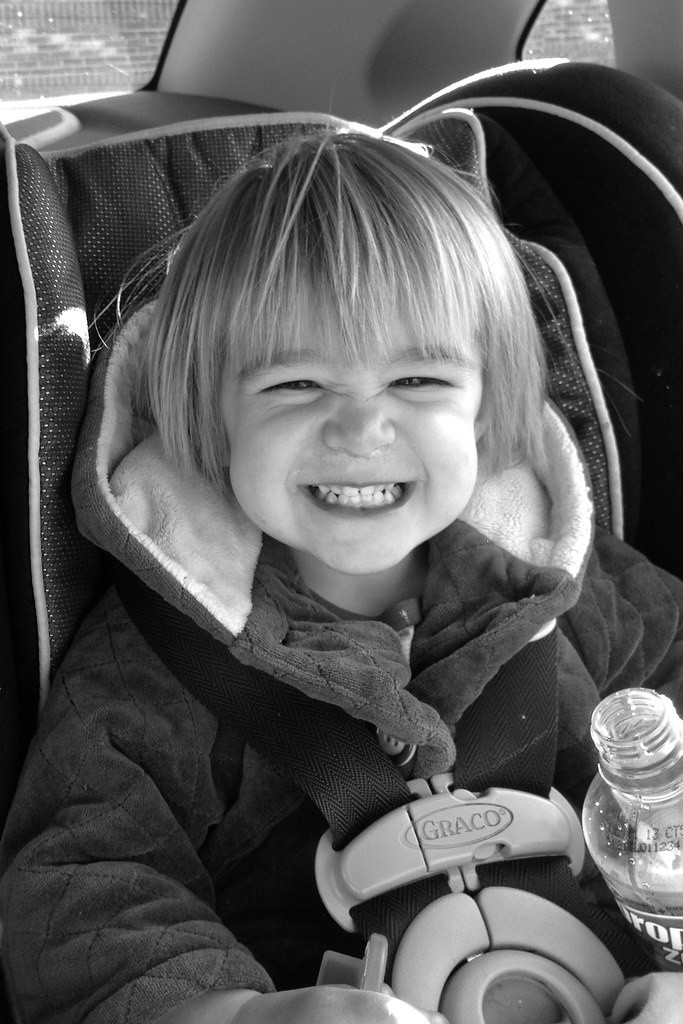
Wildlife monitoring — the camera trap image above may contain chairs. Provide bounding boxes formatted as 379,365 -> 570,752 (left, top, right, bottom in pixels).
4,49 -> 683,784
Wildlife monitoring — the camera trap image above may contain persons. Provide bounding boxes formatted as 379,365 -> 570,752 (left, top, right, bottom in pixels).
4,126 -> 679,1024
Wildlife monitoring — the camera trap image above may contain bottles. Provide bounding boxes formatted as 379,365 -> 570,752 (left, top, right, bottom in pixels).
581,683 -> 683,977
